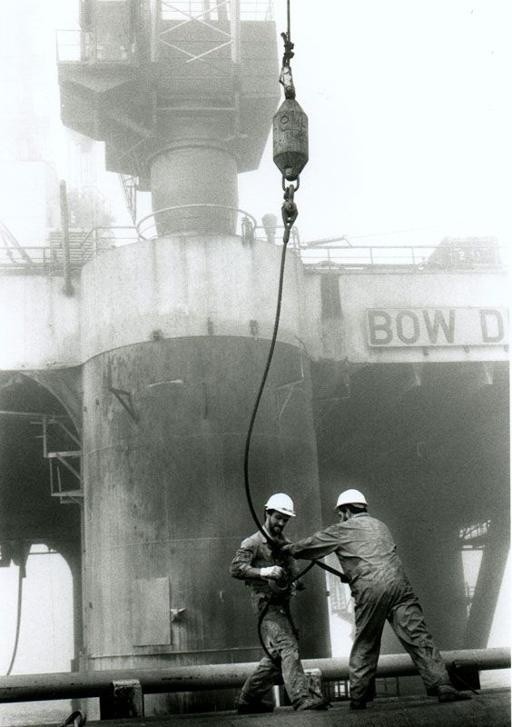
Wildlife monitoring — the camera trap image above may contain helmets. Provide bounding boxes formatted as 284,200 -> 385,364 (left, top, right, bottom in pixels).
334,488 -> 368,511
264,493 -> 296,519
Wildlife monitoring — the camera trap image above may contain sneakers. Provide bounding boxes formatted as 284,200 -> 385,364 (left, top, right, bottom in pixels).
438,684 -> 472,702
350,699 -> 368,710
238,700 -> 277,714
298,695 -> 330,709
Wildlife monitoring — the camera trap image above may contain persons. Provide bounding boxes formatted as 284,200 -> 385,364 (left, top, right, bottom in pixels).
230,493 -> 328,712
279,489 -> 472,713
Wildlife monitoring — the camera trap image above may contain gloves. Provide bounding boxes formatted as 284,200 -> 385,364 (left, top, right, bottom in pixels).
259,565 -> 284,581
